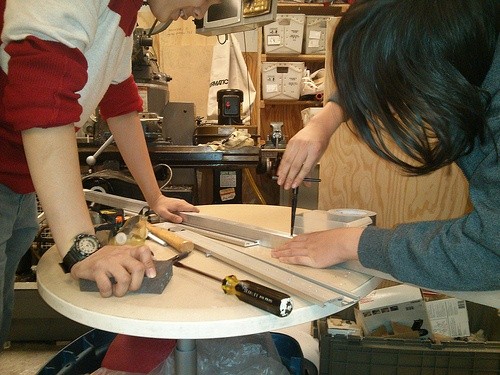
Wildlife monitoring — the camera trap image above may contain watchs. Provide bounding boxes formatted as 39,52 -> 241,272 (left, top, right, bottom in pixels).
58,234 -> 103,275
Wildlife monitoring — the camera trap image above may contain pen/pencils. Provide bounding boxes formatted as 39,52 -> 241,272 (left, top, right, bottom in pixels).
148,232 -> 167,247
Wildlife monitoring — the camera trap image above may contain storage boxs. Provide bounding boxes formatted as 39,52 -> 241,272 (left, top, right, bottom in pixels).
304,16 -> 334,55
262,62 -> 305,100
328,283 -> 470,341
264,13 -> 305,54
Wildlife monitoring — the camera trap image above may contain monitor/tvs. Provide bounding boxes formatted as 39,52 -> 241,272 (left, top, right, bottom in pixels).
203,0 -> 241,28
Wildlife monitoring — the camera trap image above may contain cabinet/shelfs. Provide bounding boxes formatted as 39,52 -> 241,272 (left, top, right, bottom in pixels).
229,0 -> 352,153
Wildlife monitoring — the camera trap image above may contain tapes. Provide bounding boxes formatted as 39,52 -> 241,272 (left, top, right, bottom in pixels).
328,207 -> 377,226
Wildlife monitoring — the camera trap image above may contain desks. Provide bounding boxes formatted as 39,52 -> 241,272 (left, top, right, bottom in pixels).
37,203 -> 383,375
72,148 -> 259,204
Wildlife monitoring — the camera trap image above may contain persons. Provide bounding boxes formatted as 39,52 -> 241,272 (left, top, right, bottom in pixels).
0,0 -> 222,347
272,0 -> 500,292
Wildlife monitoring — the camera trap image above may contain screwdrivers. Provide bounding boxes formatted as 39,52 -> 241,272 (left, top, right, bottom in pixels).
172,261 -> 292,318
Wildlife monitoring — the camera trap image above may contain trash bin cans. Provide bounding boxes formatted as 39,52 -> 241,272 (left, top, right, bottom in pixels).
162,185 -> 193,205
33,329 -> 318,375
